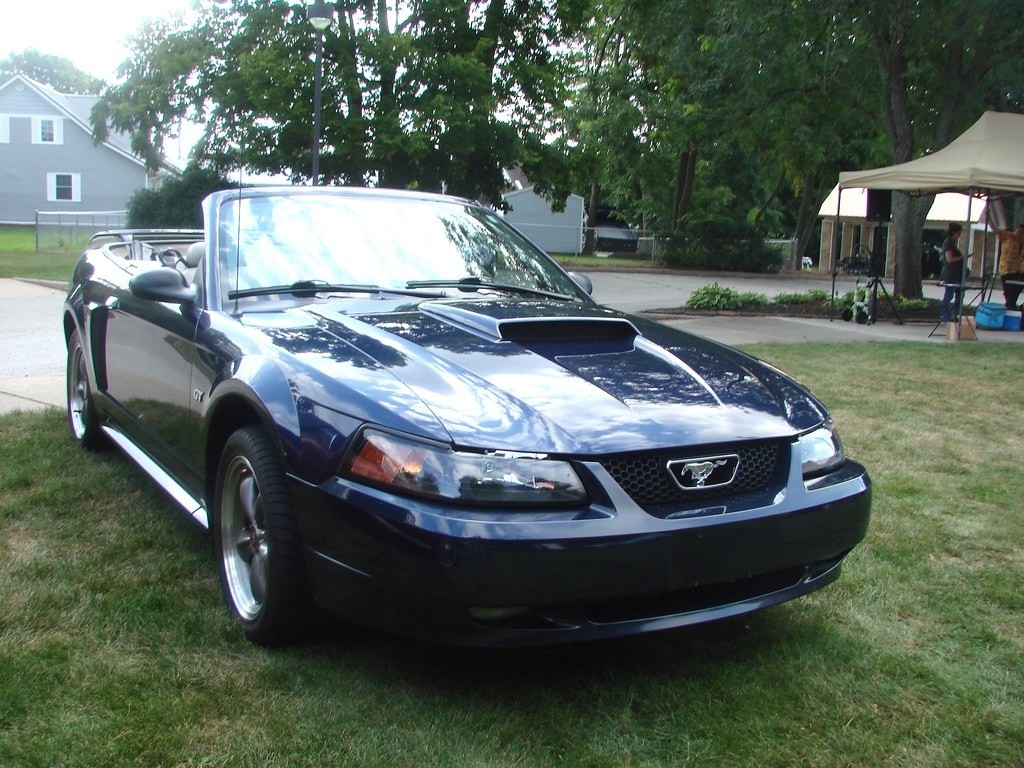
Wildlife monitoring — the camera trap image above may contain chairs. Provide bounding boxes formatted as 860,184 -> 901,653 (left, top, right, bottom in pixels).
183,244 -> 257,303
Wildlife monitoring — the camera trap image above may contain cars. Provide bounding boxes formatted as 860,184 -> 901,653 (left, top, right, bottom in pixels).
581,206 -> 638,252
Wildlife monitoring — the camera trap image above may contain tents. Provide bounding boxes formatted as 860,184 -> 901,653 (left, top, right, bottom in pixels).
830,111 -> 1024,341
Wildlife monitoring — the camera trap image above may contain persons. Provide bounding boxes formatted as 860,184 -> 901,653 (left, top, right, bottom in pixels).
941,223 -> 971,325
988,209 -> 1024,308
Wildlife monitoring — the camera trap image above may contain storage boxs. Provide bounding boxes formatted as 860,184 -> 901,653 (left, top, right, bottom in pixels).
1002,310 -> 1022,332
945,315 -> 977,341
975,302 -> 1007,330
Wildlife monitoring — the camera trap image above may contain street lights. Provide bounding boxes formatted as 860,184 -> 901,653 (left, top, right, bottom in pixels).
306,1 -> 336,186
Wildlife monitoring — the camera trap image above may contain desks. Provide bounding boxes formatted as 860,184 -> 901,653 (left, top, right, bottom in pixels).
1005,280 -> 1024,288
928,282 -> 987,341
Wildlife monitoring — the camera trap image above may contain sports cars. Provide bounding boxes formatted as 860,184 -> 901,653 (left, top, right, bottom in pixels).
62,186 -> 873,650
